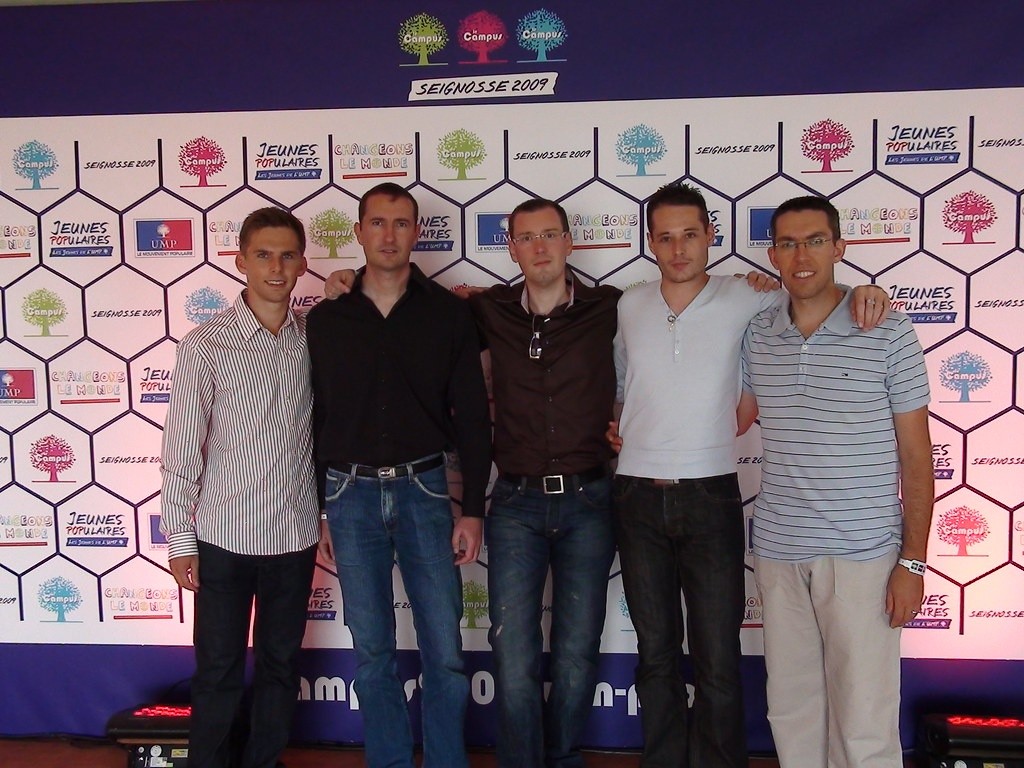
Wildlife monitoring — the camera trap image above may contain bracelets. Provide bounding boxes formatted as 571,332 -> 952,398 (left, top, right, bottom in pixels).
898,558 -> 927,576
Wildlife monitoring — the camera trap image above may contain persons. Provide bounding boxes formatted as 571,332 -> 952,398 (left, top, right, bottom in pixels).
611,183 -> 889,766
160,208 -> 492,768
606,196 -> 934,768
304,180 -> 494,768
323,197 -> 782,768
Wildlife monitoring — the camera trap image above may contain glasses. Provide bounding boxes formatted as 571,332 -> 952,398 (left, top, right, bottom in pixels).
529,312 -> 547,360
512,232 -> 567,247
774,236 -> 831,251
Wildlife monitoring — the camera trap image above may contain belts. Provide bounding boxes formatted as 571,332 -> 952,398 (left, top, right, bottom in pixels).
499,462 -> 611,494
616,472 -> 738,490
330,452 -> 448,480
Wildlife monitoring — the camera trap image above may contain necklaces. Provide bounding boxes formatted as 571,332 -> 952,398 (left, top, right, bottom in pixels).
667,306 -> 676,322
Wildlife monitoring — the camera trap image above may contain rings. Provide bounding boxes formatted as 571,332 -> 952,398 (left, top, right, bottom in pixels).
866,297 -> 875,304
911,610 -> 917,614
